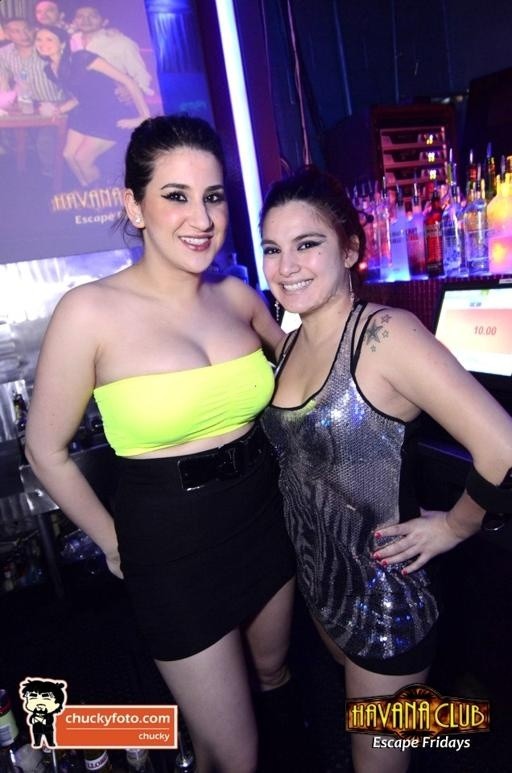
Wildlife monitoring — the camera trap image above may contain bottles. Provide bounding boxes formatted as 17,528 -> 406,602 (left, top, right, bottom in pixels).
389,142 -> 511,281
12,394 -> 29,465
0,687 -> 201,773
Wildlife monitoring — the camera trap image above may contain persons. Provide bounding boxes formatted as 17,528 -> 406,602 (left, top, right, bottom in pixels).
0,0 -> 156,186
